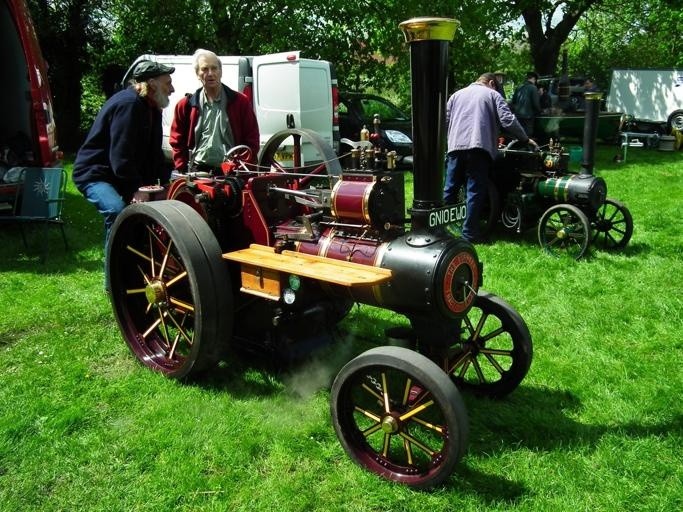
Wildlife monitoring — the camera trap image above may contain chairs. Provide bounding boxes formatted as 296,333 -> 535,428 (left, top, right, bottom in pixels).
1,168 -> 68,263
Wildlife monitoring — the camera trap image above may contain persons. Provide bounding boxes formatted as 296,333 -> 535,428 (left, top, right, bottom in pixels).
441,70 -> 553,245
72,59 -> 174,296
578,77 -> 600,111
169,48 -> 260,183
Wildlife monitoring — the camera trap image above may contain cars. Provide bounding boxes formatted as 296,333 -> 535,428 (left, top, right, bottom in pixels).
506,75 -> 586,110
335,89 -> 414,162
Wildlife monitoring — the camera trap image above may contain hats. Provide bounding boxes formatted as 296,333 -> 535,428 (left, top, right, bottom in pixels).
132,61 -> 175,83
480,72 -> 497,85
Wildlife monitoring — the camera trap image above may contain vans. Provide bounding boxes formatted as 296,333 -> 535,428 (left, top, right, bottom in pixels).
0,1 -> 64,209
116,50 -> 338,170
